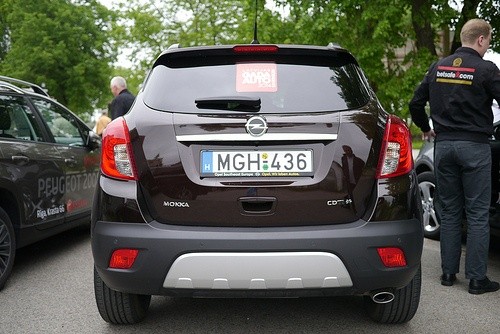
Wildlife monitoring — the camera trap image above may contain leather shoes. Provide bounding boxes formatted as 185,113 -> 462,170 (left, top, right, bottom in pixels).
441,272 -> 457,285
469,277 -> 500,295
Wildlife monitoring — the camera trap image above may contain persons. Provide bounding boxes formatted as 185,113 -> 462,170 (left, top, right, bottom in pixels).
95,75 -> 136,136
407,17 -> 500,295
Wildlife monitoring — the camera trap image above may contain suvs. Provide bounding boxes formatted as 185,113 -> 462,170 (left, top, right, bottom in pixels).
89,37 -> 425,326
0,75 -> 103,289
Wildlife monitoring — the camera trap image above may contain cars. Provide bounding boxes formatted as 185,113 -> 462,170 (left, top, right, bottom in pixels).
414,110 -> 500,238
